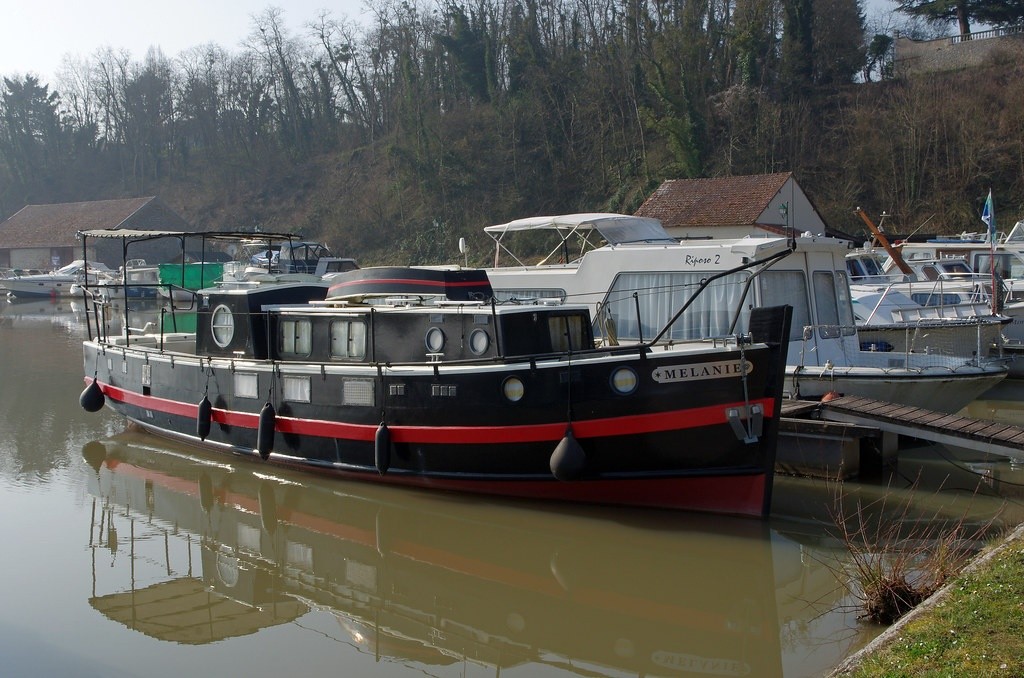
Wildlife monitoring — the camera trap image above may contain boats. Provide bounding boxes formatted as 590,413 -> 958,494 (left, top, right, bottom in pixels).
0,193 -> 1024,525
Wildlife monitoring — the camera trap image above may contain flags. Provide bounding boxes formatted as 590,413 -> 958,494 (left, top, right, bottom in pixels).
981,191 -> 998,244
